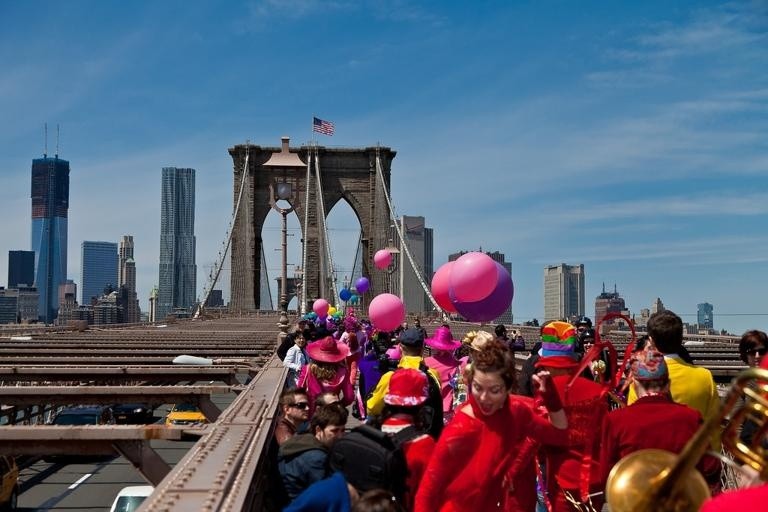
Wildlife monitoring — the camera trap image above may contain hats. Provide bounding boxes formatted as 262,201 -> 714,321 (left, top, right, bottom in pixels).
296,318 -> 306,323
384,369 -> 431,407
534,317 -> 596,369
628,351 -> 670,383
304,335 -> 351,363
424,326 -> 508,354
399,328 -> 425,348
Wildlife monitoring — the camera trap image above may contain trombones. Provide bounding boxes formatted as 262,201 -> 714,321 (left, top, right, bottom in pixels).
606,368 -> 768,512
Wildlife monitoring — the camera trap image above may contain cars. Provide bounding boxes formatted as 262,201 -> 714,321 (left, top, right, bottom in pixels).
111,404 -> 155,421
109,484 -> 154,511
0,454 -> 20,512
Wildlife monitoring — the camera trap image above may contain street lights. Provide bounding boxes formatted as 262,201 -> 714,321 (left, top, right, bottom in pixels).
341,273 -> 349,307
171,355 -> 259,380
260,135 -> 310,351
349,284 -> 358,316
383,238 -> 399,295
291,264 -> 304,327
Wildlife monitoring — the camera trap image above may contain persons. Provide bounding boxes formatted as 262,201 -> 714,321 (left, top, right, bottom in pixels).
624,309 -> 723,453
273,318 -> 656,512
739,329 -> 767,368
596,350 -> 722,496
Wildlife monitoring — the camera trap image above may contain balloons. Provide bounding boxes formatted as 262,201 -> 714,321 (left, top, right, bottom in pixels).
354,275 -> 370,296
338,288 -> 351,301
311,298 -> 344,324
350,294 -> 357,303
429,251 -> 514,323
372,250 -> 391,270
368,293 -> 405,333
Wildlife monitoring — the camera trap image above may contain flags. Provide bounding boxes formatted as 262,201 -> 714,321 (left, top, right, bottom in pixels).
310,115 -> 335,145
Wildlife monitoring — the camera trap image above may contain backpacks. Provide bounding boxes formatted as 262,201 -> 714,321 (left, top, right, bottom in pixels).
321,419 -> 426,508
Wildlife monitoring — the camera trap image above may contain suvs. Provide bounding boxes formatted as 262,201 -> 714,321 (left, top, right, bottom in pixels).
165,402 -> 207,426
41,404 -> 117,463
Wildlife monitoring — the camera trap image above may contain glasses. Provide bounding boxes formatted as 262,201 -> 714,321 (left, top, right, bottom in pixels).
290,402 -> 308,409
746,348 -> 767,355
299,322 -> 306,325
